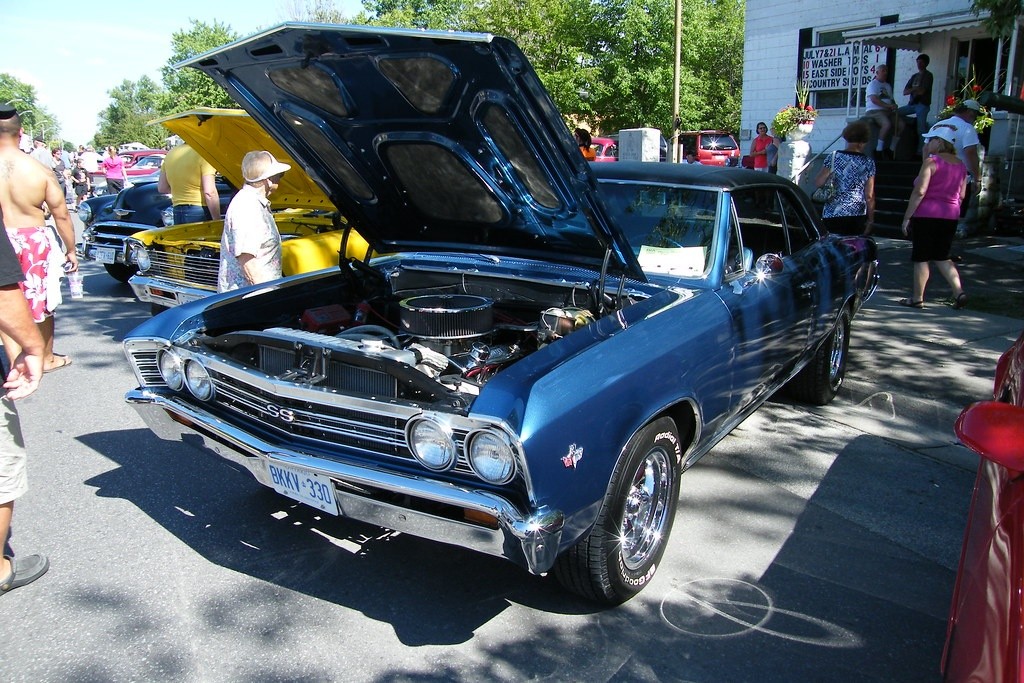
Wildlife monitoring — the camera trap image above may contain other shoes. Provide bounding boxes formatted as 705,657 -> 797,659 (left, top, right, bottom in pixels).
885,147 -> 895,162
873,147 -> 884,162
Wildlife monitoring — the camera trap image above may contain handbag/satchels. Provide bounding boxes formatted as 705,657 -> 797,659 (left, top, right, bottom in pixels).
959,171 -> 973,218
811,151 -> 835,206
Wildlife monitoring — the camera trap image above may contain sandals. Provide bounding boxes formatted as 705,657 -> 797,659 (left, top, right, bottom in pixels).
951,292 -> 967,309
897,297 -> 924,309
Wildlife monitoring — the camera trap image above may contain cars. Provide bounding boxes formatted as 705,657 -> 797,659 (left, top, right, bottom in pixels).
74,140 -> 240,285
589,136 -> 619,163
939,325 -> 1023,683
118,19 -> 882,608
606,132 -> 667,163
120,106 -> 378,314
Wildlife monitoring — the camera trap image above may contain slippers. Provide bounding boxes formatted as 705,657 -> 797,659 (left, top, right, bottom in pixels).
41,355 -> 73,374
0,553 -> 50,596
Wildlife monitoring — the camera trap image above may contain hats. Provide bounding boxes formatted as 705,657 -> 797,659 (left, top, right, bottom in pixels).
33,135 -> 44,142
960,99 -> 985,116
242,151 -> 292,183
922,127 -> 956,144
687,150 -> 696,155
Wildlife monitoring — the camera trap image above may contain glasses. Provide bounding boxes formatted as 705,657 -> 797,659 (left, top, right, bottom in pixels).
759,127 -> 766,129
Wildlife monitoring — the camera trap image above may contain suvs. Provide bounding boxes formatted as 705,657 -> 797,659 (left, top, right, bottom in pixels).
664,128 -> 740,168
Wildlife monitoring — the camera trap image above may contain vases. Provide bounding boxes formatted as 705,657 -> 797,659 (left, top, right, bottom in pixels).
789,120 -> 813,141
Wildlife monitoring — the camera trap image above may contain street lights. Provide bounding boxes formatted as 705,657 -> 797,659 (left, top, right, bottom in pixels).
42,126 -> 55,142
30,120 -> 48,152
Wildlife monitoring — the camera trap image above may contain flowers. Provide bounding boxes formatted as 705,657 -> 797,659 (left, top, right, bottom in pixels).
939,61 -> 1014,133
771,86 -> 819,140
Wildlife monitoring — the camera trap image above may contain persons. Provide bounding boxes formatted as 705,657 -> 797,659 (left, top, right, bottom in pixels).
102,146 -> 128,195
899,127 -> 968,308
897,54 -> 933,161
70,158 -> 91,211
922,100 -> 985,261
158,142 -> 220,225
0,105 -> 78,372
575,128 -> 596,161
765,123 -> 786,175
865,64 -> 905,160
217,151 -> 291,294
0,205 -> 49,596
815,120 -> 876,237
0,136 -> 103,219
750,122 -> 773,172
